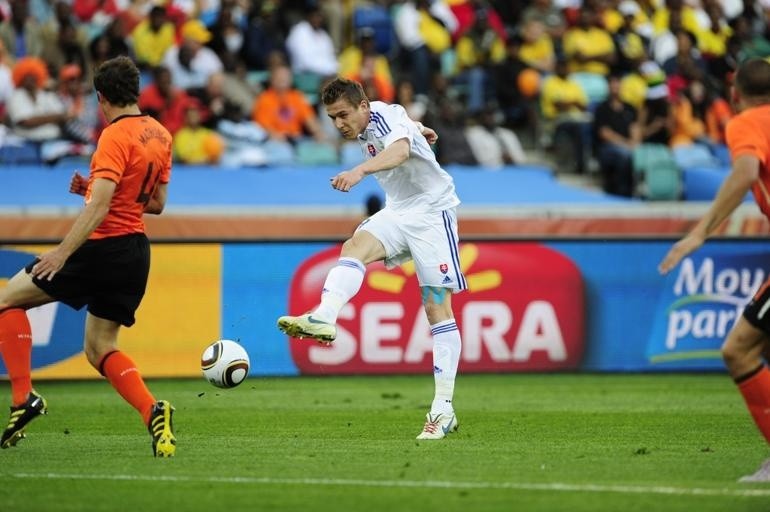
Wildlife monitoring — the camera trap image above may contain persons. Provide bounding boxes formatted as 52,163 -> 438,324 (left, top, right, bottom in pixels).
656,59 -> 770,481
276,76 -> 470,440
1,0 -> 770,203
1,55 -> 177,459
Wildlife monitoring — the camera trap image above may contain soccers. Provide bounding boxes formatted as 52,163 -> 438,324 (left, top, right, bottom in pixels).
202,339 -> 250,388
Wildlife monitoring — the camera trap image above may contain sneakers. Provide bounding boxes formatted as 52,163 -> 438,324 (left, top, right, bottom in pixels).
277,311 -> 337,346
416,411 -> 458,440
1,388 -> 49,449
148,399 -> 177,457
